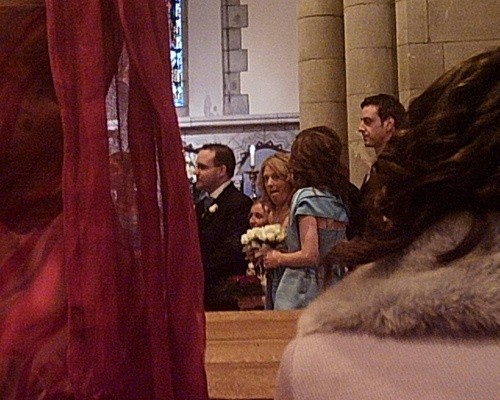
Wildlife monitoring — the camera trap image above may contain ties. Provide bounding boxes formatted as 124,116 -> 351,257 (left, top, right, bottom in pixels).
200,197 -> 214,219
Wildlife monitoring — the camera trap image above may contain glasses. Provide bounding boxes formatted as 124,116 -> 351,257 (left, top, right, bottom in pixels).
194,163 -> 222,171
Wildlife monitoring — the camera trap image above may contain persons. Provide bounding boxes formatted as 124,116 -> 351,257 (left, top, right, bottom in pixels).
247,194 -> 271,230
189,142 -> 252,312
263,127 -> 362,313
259,152 -> 297,309
106,152 -> 176,290
276,48 -> 500,400
346,94 -> 412,270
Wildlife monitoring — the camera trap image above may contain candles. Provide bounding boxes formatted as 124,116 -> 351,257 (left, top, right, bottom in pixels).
250,144 -> 256,166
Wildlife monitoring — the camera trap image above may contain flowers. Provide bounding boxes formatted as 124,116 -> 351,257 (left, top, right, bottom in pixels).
241,223 -> 288,279
